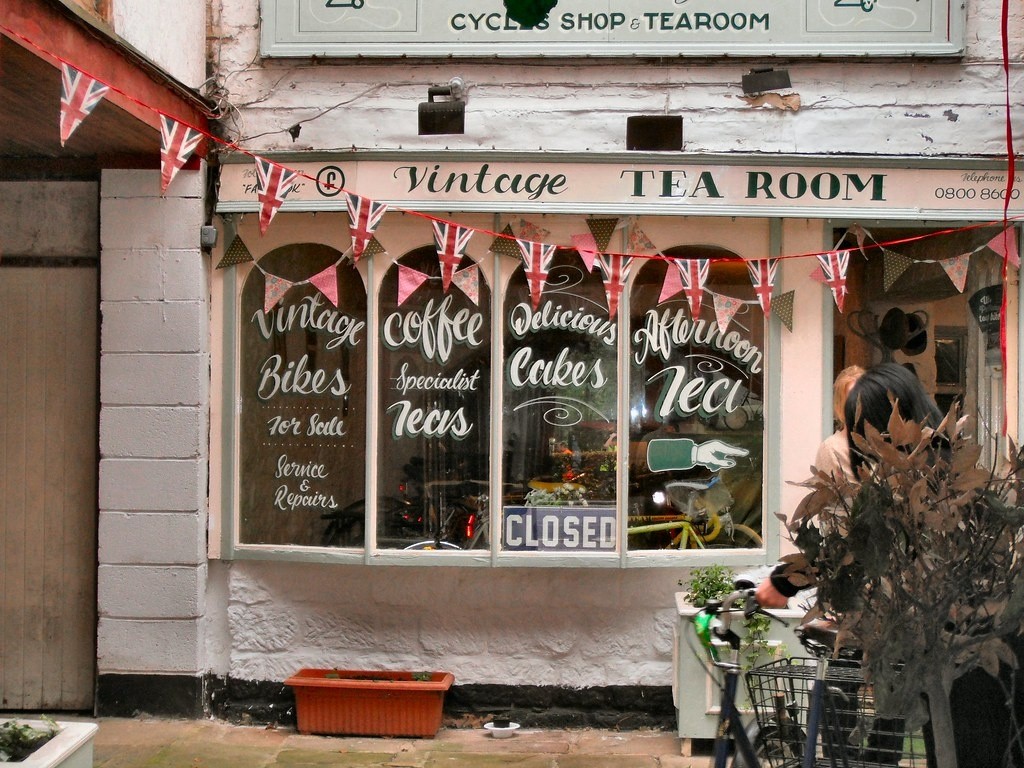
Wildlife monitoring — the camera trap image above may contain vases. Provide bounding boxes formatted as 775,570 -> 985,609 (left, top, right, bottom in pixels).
493,715 -> 511,728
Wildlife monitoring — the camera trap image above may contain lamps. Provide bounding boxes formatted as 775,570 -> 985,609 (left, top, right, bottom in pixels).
741,67 -> 792,94
417,78 -> 466,135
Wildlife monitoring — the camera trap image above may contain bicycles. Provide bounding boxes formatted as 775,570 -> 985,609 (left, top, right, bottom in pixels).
320,438 -> 762,553
683,578 -> 934,768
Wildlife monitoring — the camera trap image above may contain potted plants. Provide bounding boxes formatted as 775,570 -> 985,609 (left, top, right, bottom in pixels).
283,669 -> 454,740
0,713 -> 99,768
673,559 -> 841,756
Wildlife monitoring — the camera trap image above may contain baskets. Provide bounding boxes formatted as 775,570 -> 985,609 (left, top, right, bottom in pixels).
745,657 -> 929,768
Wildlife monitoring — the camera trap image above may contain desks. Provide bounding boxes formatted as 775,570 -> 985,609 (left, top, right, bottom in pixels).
666,430 -> 763,448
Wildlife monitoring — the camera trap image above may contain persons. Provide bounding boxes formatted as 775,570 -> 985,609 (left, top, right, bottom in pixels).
754,362 -> 1024,768
